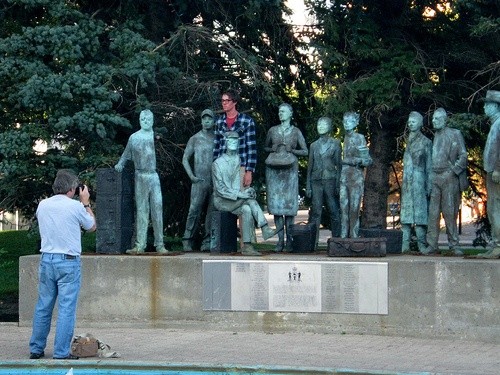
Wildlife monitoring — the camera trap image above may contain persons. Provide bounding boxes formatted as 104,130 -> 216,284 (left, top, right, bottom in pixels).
28,168 -> 97,359
113,109 -> 171,256
400,111 -> 433,255
475,89 -> 500,259
339,109 -> 373,239
306,116 -> 342,250
210,130 -> 285,256
421,106 -> 469,256
211,89 -> 257,249
180,108 -> 215,252
263,102 -> 310,253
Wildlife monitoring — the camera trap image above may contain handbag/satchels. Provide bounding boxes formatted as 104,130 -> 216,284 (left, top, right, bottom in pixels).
72,333 -> 122,358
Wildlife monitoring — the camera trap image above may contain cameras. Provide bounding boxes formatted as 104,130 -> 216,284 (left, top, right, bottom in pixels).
74,184 -> 97,203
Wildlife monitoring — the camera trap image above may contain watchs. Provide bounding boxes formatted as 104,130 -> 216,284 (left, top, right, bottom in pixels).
84,203 -> 92,207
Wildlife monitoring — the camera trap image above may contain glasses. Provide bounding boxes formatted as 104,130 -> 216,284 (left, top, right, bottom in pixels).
221,98 -> 232,103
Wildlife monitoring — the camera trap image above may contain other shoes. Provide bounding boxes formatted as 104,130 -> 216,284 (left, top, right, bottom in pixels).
29,351 -> 45,358
52,354 -> 79,359
